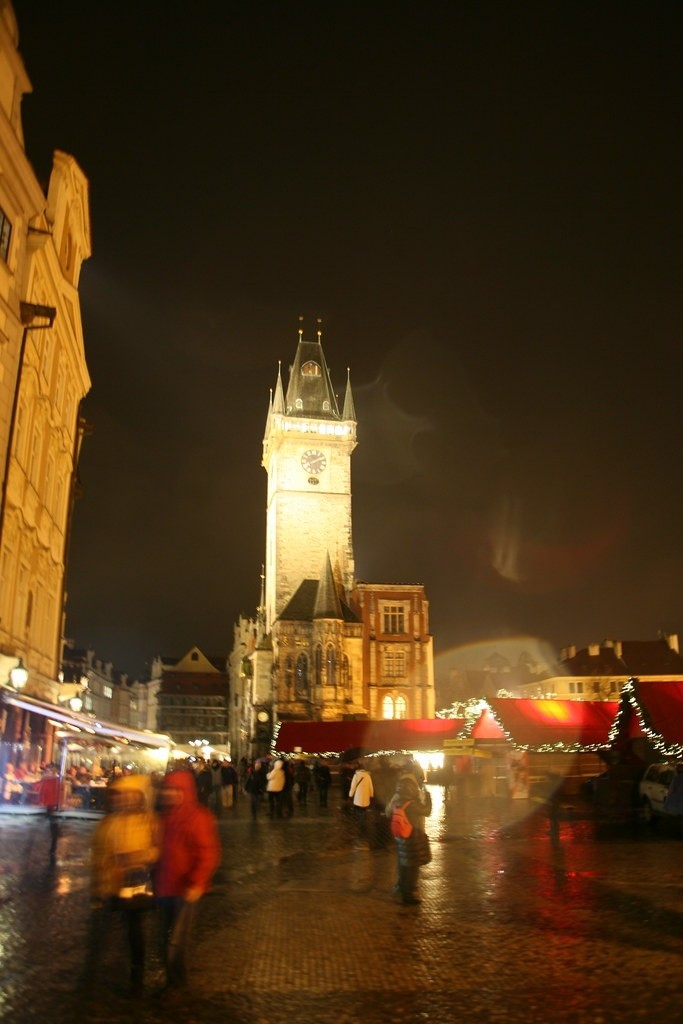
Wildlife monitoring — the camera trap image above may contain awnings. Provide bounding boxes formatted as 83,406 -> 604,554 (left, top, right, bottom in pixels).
3,689 -> 175,749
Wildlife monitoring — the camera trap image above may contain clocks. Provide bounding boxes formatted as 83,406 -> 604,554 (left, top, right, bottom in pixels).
301,450 -> 327,474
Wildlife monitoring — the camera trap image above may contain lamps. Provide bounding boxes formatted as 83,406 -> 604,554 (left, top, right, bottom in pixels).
9,657 -> 30,695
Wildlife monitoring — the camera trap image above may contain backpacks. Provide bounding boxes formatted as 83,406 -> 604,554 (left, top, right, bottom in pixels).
391,800 -> 416,839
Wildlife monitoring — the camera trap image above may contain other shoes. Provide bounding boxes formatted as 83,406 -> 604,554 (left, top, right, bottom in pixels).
404,896 -> 422,904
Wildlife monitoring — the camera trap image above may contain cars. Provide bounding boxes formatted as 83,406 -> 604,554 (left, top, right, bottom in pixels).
635,761 -> 683,828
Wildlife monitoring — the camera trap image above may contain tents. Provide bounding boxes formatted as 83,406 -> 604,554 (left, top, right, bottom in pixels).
470,678 -> 683,812
269,717 -> 465,800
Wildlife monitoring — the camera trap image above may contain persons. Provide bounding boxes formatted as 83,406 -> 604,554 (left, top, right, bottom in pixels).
1,753 -> 430,839
76,771 -> 162,1001
153,769 -> 222,994
384,777 -> 431,905
